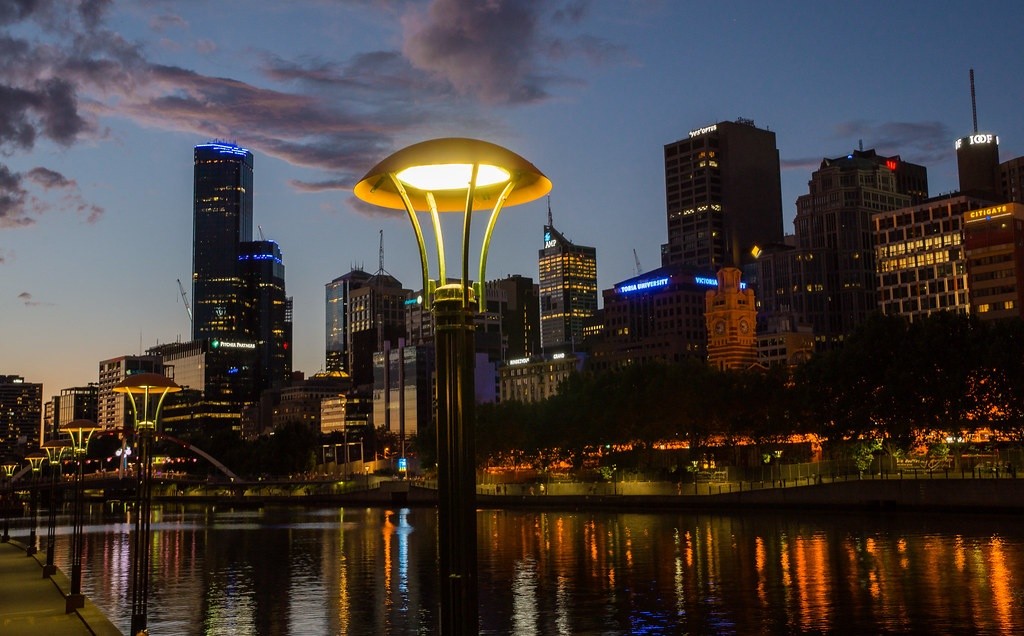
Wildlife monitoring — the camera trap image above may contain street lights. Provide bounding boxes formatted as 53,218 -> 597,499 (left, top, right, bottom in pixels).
1,420 -> 104,615
353,137 -> 553,636
113,373 -> 182,635
